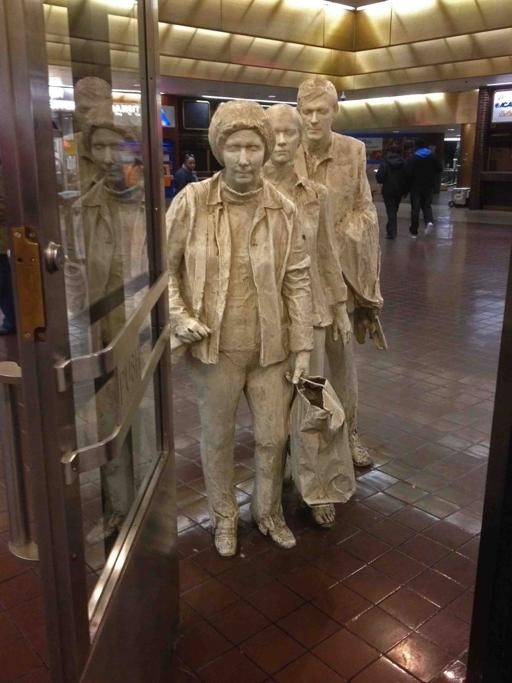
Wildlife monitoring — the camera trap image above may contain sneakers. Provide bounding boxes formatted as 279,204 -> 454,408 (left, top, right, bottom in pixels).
424,221 -> 433,235
408,231 -> 416,238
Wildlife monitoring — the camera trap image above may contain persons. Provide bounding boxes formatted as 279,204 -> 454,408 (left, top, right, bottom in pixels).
294,78 -> 388,469
432,146 -> 445,196
375,142 -> 409,239
165,98 -> 315,559
405,137 -> 440,239
57,77 -> 113,352
262,103 -> 356,531
171,153 -> 200,193
68,98 -> 157,546
